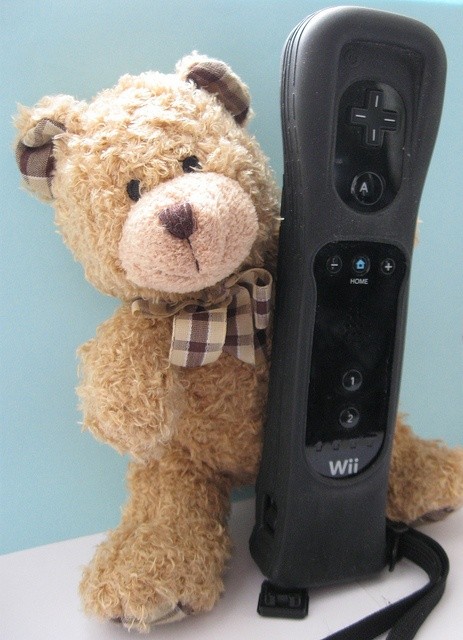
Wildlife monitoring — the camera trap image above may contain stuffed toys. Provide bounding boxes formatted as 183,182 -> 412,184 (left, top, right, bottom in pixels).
14,52 -> 462,632
